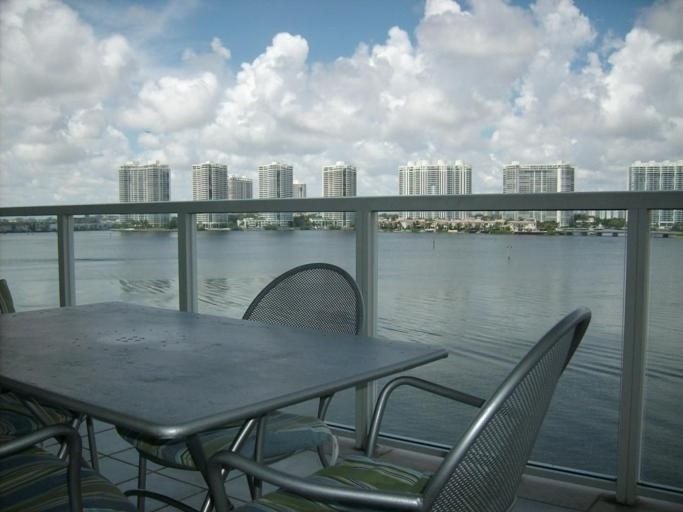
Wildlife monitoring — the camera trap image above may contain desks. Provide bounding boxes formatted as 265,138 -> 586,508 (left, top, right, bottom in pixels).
0,301 -> 448,511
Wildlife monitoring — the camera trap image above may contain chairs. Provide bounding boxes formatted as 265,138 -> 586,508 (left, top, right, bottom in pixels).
0,280 -> 90,469
0,423 -> 136,512
206,306 -> 591,512
114,262 -> 363,509
